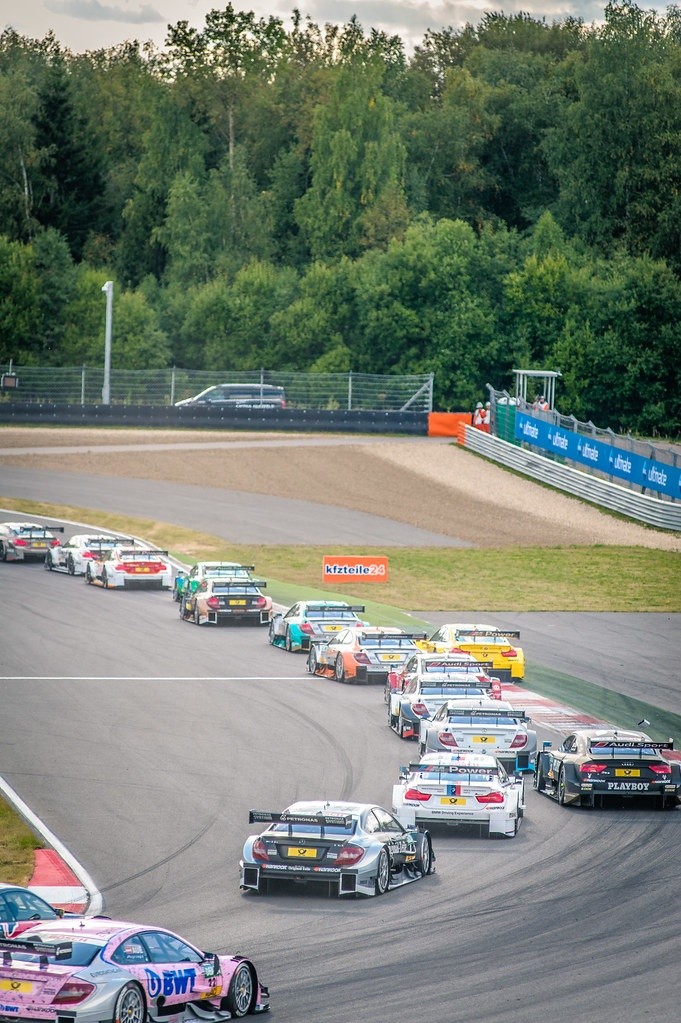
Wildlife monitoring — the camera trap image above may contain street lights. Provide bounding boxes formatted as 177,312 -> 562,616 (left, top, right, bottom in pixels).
102,281 -> 114,404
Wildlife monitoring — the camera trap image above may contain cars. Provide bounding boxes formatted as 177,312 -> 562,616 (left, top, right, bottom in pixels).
238,801 -> 436,898
0,522 -> 64,562
44,535 -> 134,576
0,884 -> 271,1023
179,576 -> 273,627
412,624 -> 525,683
175,384 -> 287,410
384,652 -> 501,699
306,627 -> 423,684
172,562 -> 254,602
532,730 -> 681,810
388,673 -> 493,741
267,601 -> 369,652
393,751 -> 526,838
85,547 -> 172,591
419,698 -> 537,772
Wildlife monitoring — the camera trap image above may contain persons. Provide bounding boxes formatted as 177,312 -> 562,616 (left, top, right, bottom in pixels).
473,402 -> 490,432
533,396 -> 548,412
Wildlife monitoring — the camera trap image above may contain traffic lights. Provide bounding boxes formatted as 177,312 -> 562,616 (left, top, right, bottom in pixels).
1,375 -> 19,389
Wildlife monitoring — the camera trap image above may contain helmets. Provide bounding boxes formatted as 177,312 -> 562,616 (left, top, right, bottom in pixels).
485,401 -> 490,407
476,402 -> 484,409
538,396 -> 545,399
